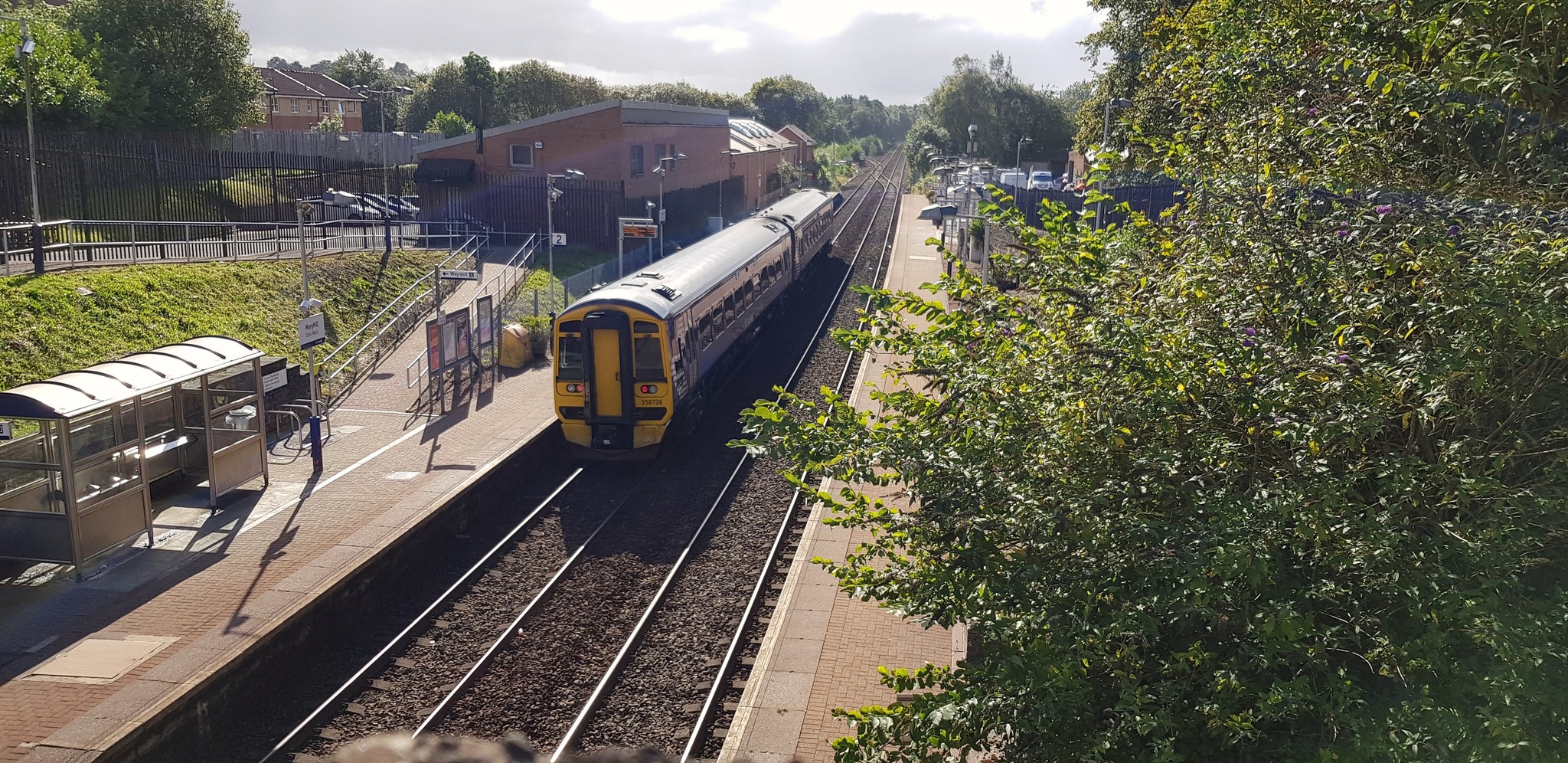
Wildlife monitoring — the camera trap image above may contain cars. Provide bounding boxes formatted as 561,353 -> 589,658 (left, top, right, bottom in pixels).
304,193 -> 420,224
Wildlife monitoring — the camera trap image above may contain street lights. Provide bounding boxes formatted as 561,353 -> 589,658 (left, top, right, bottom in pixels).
1095,97 -> 1134,234
654,153 -> 687,257
12,11 -> 41,223
547,170 -> 587,318
832,127 -> 836,166
1014,138 -> 1033,208
347,84 -> 415,253
718,150 -> 731,217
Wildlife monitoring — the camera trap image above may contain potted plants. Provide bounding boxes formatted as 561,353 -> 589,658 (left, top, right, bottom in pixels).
519,315 -> 552,356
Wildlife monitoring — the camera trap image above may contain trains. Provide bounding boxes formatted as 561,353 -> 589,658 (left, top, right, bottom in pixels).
551,187 -> 835,467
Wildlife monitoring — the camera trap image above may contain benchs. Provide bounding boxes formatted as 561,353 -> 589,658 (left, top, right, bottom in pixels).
74,469 -> 139,505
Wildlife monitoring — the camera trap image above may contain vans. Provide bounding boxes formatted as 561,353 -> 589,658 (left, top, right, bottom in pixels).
1031,172 -> 1054,191
